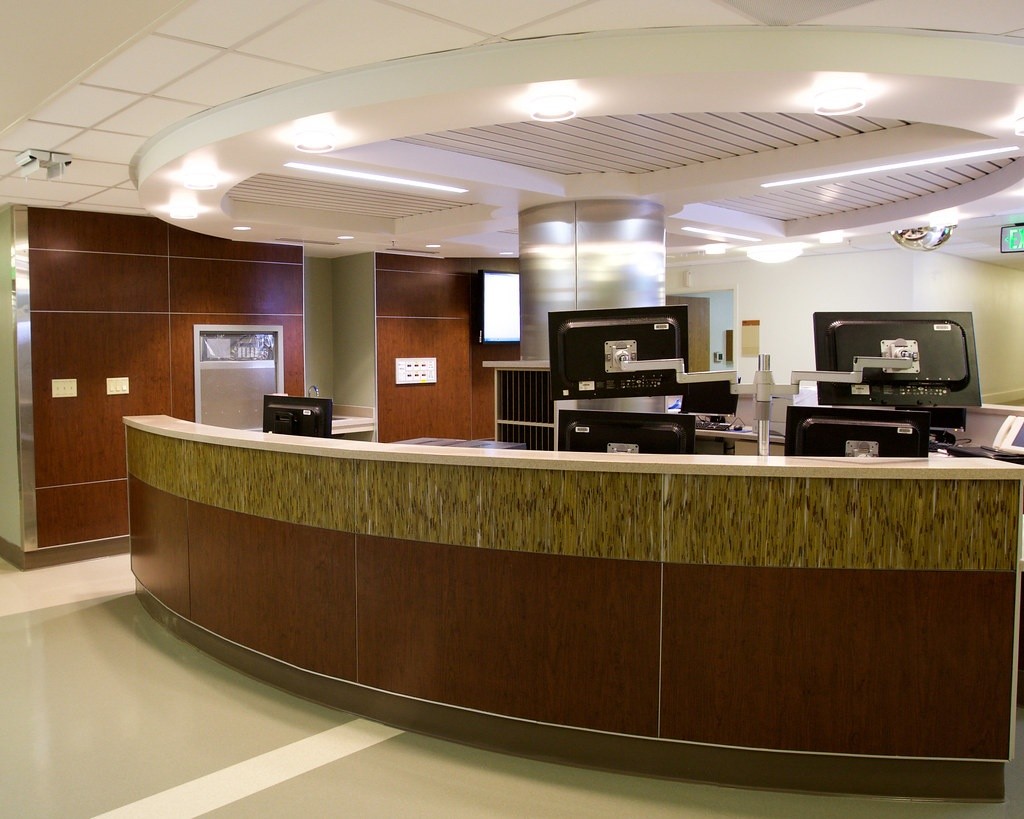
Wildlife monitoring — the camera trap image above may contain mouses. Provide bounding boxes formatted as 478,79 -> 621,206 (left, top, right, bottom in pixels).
734,424 -> 742,431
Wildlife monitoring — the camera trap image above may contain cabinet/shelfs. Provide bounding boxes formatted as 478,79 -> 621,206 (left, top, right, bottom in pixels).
374,251 -> 472,442
0,203 -> 173,572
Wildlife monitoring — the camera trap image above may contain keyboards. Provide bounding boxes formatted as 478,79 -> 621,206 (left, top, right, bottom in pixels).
928,442 -> 949,452
695,422 -> 731,431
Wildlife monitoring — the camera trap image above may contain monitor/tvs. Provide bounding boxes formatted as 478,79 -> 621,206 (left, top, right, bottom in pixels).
558,409 -> 695,455
681,377 -> 740,422
785,312 -> 982,458
548,304 -> 689,400
477,269 -> 520,344
263,395 -> 333,439
